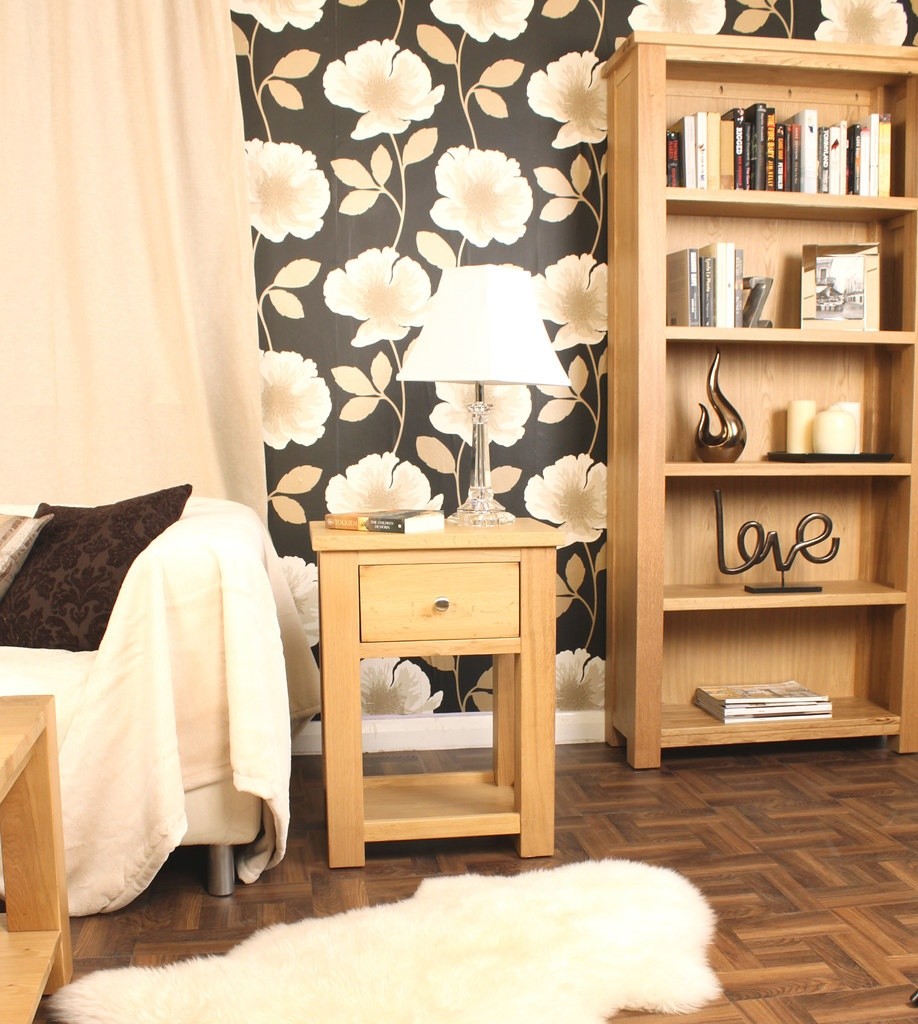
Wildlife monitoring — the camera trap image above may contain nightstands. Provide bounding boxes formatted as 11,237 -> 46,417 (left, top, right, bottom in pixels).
309,517 -> 566,867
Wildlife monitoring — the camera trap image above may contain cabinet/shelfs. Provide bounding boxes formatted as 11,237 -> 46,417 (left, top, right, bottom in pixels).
598,35 -> 918,769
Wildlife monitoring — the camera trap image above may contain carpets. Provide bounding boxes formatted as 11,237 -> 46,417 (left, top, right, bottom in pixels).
44,858 -> 724,1024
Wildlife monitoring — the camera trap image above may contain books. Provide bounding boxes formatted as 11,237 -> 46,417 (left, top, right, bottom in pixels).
665,103 -> 891,197
694,680 -> 832,724
666,242 -> 743,327
325,509 -> 444,534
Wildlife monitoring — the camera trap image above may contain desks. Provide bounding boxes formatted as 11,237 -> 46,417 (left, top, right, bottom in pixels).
0,695 -> 73,1024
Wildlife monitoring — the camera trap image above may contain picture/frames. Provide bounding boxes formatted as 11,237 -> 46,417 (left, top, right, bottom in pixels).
801,242 -> 879,330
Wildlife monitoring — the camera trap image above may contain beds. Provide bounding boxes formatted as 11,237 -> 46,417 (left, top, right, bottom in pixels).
0,484 -> 323,919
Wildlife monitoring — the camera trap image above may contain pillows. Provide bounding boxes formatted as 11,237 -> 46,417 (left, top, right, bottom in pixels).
0,513 -> 55,600
0,482 -> 192,653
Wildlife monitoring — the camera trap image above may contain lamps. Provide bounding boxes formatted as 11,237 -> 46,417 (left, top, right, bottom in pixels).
397,265 -> 572,525
